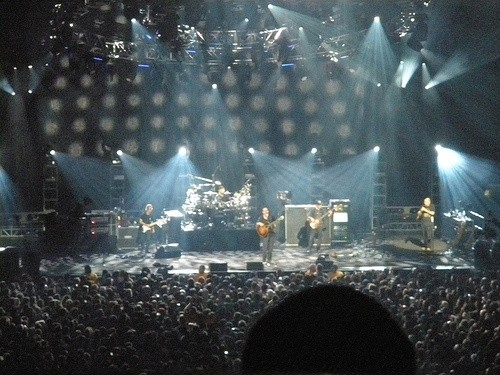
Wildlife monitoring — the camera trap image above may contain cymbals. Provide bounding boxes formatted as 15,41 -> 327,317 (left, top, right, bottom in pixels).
202,192 -> 218,195
223,192 -> 234,195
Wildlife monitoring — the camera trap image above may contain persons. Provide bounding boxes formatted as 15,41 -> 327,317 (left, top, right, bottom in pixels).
241,285 -> 417,375
418,198 -> 435,242
137,204 -> 156,255
306,200 -> 329,253
256,207 -> 279,266
280,255 -> 500,375
0,265 -> 280,375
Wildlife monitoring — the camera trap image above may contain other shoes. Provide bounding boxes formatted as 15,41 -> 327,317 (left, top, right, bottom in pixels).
145,250 -> 151,253
422,247 -> 426,251
317,248 -> 321,252
306,249 -> 310,253
427,248 -> 430,251
263,261 -> 270,266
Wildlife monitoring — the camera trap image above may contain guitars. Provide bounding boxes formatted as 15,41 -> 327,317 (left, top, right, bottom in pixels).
308,203 -> 344,229
143,218 -> 166,232
256,215 -> 285,237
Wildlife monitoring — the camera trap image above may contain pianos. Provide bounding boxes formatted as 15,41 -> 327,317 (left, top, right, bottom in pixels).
440,210 -> 473,249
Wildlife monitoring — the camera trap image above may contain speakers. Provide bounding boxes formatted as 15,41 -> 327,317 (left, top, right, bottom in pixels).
316,261 -> 333,269
247,262 -> 264,271
117,225 -> 140,249
209,263 -> 227,270
156,245 -> 182,258
